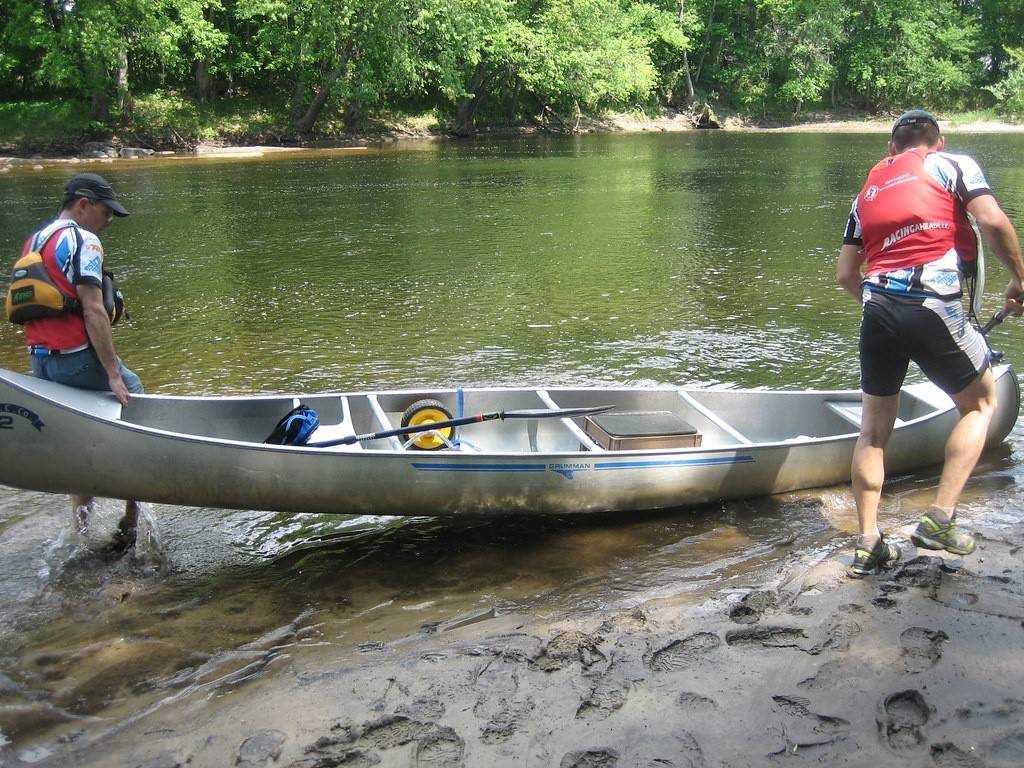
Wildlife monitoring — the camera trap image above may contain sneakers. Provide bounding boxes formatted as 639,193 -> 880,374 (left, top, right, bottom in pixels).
910,512 -> 975,555
851,531 -> 899,574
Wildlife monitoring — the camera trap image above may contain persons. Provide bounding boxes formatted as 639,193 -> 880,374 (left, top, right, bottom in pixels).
19,173 -> 145,524
837,108 -> 1024,578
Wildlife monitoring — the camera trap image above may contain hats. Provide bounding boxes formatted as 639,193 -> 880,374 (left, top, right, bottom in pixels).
892,109 -> 939,134
64,173 -> 130,217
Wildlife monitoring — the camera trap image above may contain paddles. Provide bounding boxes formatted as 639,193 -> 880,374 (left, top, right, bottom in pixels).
304,400 -> 621,449
980,306 -> 1011,338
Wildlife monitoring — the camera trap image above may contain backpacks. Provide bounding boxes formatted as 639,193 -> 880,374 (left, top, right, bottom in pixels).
262,404 -> 319,447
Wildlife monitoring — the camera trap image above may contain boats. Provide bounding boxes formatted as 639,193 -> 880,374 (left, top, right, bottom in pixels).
0,358 -> 1021,522
